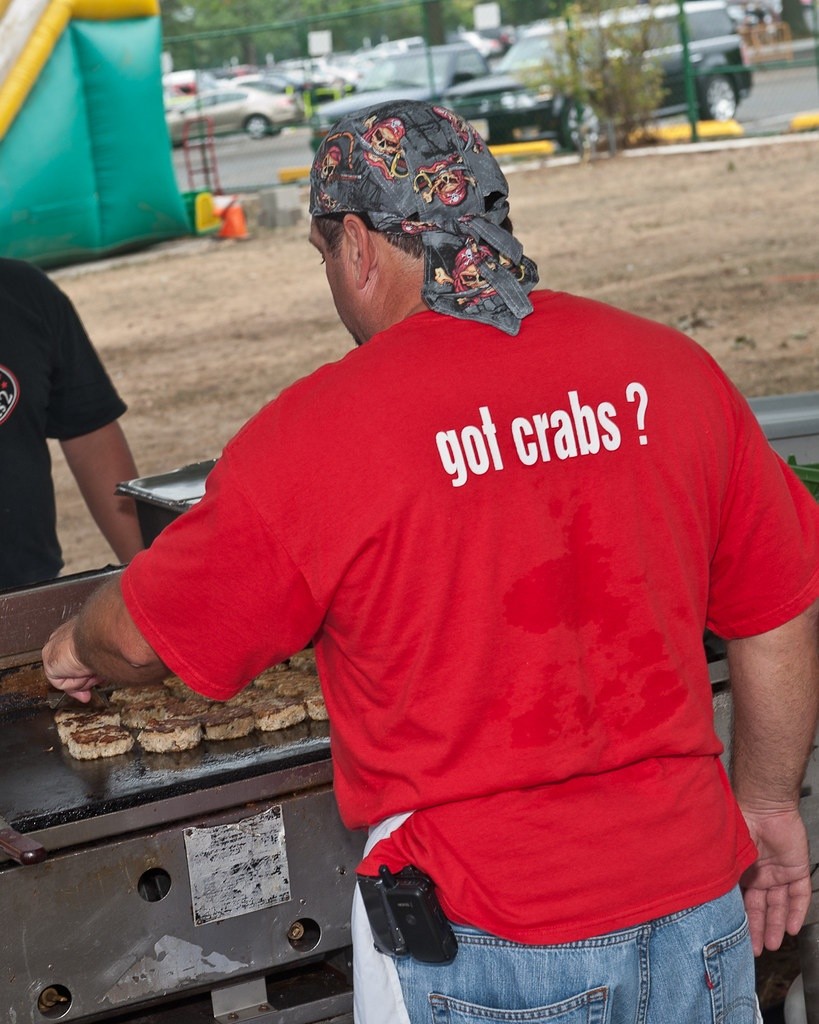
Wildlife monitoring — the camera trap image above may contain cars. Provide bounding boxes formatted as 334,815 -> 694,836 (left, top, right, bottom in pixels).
159,85 -> 305,147
153,7 -> 510,108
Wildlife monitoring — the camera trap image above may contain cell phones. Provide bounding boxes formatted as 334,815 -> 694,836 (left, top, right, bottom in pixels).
355,865 -> 459,966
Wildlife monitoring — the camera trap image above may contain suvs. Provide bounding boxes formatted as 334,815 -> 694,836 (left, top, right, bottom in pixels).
308,38 -> 490,162
439,0 -> 755,154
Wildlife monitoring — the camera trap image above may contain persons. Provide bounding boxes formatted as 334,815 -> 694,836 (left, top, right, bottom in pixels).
0,259 -> 145,598
39,101 -> 818,1024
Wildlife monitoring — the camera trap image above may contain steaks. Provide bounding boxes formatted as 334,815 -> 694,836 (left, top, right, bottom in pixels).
56,647 -> 334,760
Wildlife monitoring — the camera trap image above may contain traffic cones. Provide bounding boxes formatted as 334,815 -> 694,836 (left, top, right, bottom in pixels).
207,194 -> 254,245
